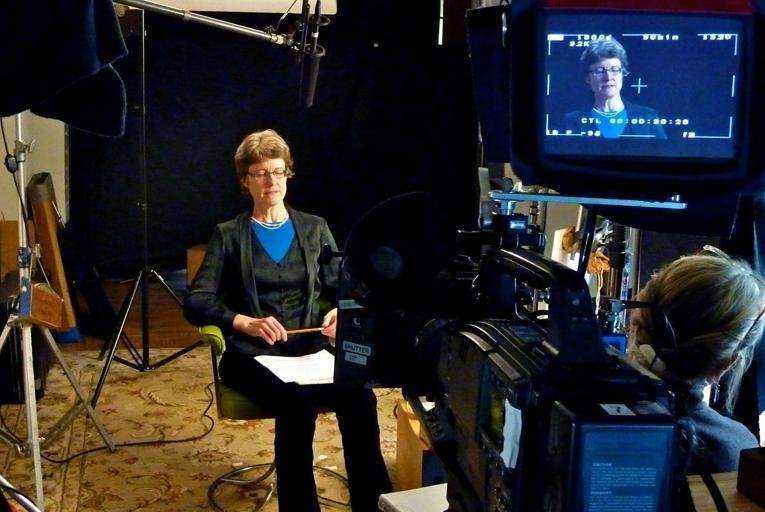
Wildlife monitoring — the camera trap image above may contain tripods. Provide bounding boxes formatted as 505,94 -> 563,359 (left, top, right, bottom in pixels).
0,114 -> 116,512
84,0 -> 292,421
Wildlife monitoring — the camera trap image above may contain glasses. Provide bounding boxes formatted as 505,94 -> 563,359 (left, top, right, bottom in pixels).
246,169 -> 286,182
589,66 -> 624,77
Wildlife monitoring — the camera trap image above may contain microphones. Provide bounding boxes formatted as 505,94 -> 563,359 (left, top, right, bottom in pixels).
298,0 -> 327,109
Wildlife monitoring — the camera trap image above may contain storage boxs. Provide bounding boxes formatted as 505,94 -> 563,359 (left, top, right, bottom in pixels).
186,244 -> 209,287
395,399 -> 436,492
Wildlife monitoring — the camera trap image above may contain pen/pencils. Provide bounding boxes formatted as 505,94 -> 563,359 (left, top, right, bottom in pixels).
287,327 -> 321,333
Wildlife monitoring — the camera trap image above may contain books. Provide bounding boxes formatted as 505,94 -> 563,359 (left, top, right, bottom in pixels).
252,348 -> 335,386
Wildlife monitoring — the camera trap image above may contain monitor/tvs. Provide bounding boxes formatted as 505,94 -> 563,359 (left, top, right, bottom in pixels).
510,1 -> 764,197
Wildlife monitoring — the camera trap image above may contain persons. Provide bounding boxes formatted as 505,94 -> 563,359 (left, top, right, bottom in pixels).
559,36 -> 668,140
184,128 -> 396,512
623,243 -> 764,476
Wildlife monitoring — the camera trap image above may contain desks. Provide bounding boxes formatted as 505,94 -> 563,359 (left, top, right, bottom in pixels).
378,482 -> 451,512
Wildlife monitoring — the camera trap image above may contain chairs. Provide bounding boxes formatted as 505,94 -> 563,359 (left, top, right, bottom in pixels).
198,324 -> 352,512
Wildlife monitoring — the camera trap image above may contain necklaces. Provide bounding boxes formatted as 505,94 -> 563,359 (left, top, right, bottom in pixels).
591,107 -> 626,118
250,216 -> 291,231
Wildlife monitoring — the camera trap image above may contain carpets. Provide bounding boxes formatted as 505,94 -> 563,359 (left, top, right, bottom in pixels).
0,345 -> 407,512
